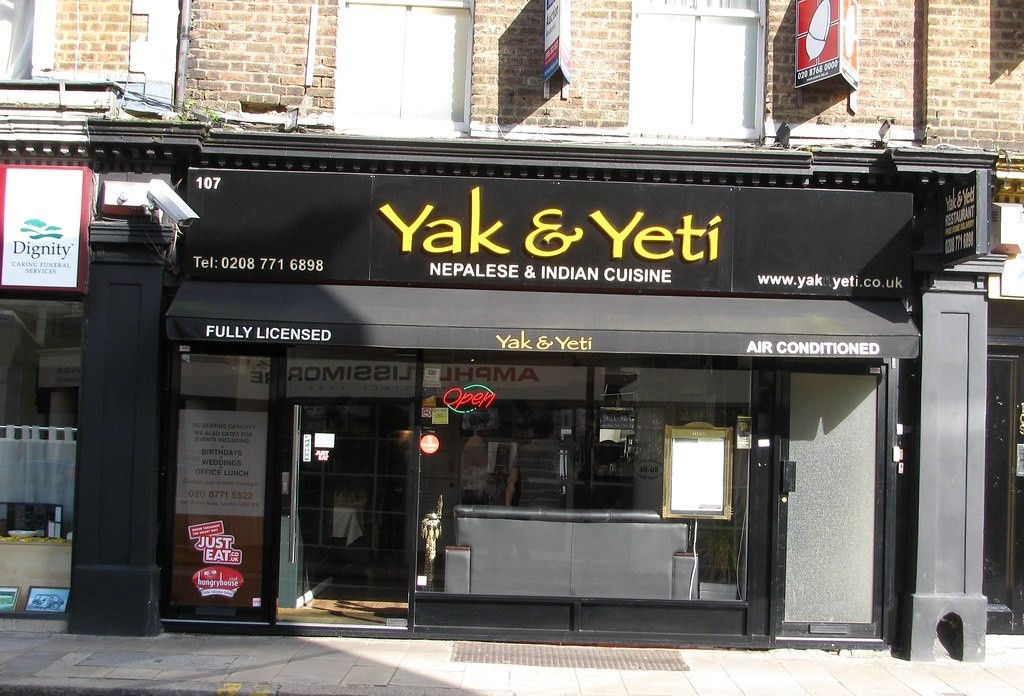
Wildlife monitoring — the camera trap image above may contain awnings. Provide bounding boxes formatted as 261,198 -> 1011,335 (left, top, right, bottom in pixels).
165,281 -> 920,360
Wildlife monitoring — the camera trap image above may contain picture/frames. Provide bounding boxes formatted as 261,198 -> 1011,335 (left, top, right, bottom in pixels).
0,586 -> 21,611
24,586 -> 71,612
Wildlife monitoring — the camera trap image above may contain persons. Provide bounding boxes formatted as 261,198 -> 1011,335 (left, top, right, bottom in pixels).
457,410 -> 579,506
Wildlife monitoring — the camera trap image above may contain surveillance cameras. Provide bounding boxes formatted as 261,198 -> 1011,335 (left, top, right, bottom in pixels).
147,179 -> 200,228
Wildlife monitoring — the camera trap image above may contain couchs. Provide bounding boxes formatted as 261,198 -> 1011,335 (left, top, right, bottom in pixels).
445,505 -> 699,600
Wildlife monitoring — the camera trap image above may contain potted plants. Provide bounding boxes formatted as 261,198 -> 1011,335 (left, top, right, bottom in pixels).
699,519 -> 737,601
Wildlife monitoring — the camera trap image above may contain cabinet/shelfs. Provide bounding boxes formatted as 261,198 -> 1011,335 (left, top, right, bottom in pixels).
282,397 -> 746,580
